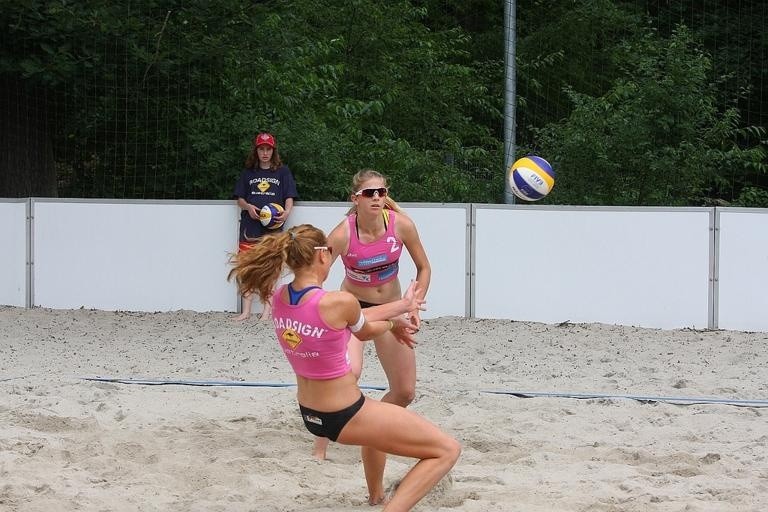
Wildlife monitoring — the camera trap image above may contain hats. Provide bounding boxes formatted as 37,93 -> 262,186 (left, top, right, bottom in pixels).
255,132 -> 274,147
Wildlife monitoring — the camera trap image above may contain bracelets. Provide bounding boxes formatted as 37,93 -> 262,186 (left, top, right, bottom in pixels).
386,318 -> 397,333
246,203 -> 251,210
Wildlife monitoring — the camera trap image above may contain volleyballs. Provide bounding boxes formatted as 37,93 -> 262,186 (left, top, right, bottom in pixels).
509,155 -> 555,202
260,203 -> 286,230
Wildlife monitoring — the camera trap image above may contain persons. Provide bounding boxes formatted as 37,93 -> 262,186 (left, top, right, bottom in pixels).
231,133 -> 300,319
226,223 -> 462,512
311,167 -> 432,460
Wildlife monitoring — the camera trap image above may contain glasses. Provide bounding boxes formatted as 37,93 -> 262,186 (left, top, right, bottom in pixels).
355,186 -> 389,199
